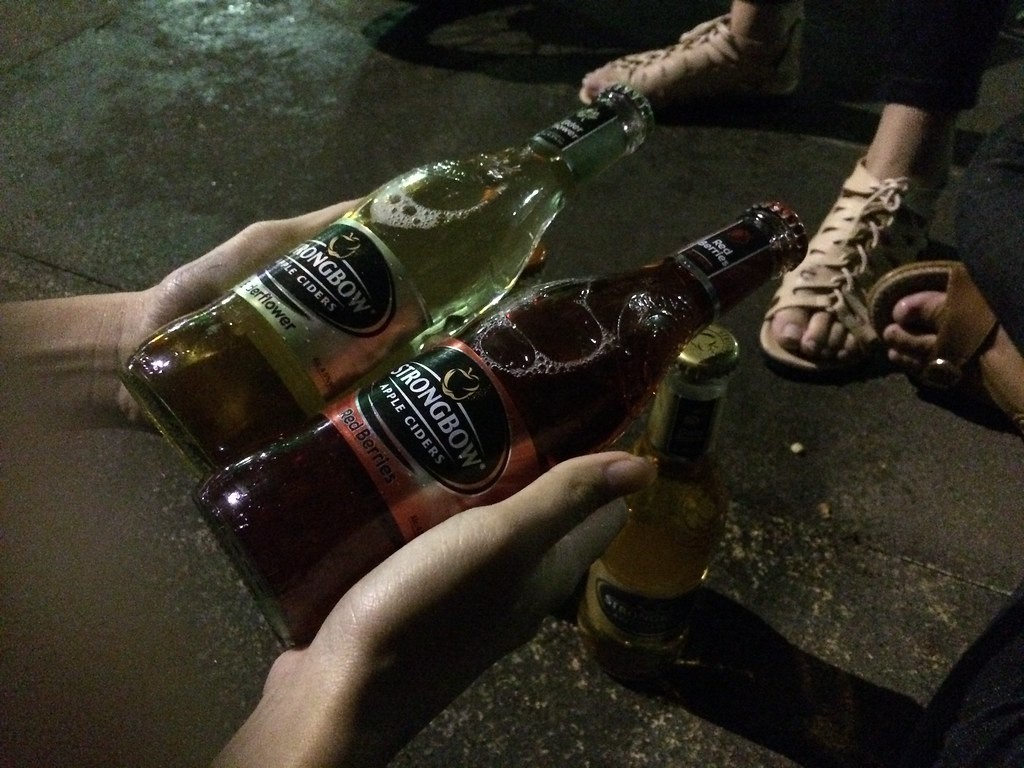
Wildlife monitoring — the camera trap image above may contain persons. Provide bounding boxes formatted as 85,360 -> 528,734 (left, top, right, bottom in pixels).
0,196 -> 655,768
870,113 -> 1023,434
580,1 -> 1024,370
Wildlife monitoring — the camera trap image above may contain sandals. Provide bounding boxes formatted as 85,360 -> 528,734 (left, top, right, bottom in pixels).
761,156 -> 942,370
578,10 -> 787,106
867,259 -> 1024,435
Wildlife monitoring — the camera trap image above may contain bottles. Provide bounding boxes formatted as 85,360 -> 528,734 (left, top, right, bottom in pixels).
191,201 -> 809,649
120,81 -> 657,478
576,324 -> 742,682
